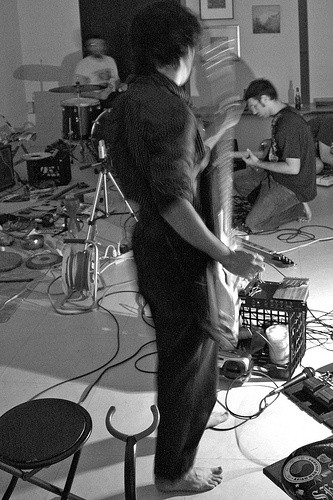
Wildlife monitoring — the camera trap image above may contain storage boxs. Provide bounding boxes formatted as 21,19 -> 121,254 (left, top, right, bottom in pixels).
27,152 -> 72,186
239,281 -> 307,380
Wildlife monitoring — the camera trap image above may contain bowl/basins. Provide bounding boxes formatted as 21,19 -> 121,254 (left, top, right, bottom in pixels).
21,234 -> 44,250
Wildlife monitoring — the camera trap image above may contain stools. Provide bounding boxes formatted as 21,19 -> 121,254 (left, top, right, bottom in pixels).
0,398 -> 93,500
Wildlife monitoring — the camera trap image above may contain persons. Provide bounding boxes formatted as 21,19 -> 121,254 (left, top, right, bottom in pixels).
108,0 -> 264,492
72,35 -> 119,100
242,79 -> 317,231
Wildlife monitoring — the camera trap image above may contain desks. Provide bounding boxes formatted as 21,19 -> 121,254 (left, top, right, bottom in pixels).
198,104 -> 333,115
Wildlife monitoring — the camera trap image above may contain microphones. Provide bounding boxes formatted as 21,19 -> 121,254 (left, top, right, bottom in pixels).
267,367 -> 315,397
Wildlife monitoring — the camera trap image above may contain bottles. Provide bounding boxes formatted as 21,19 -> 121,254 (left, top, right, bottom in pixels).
294,87 -> 301,110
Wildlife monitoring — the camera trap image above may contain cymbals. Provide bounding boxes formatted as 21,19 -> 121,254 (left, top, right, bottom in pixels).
49,85 -> 107,93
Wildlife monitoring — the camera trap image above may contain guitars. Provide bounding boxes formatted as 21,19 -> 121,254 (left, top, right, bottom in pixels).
226,139 -> 272,172
232,229 -> 295,266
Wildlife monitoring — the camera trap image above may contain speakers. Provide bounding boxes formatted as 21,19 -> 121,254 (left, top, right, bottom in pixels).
0,144 -> 15,192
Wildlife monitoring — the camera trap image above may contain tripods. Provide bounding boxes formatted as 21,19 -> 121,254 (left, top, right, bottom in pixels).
84,139 -> 138,254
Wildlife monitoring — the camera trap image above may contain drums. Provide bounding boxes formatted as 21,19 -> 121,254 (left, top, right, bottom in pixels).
61,98 -> 101,139
91,109 -> 113,160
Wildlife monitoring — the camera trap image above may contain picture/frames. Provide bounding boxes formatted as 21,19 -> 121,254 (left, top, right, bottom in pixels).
200,24 -> 241,62
252,5 -> 280,33
199,0 -> 234,21
